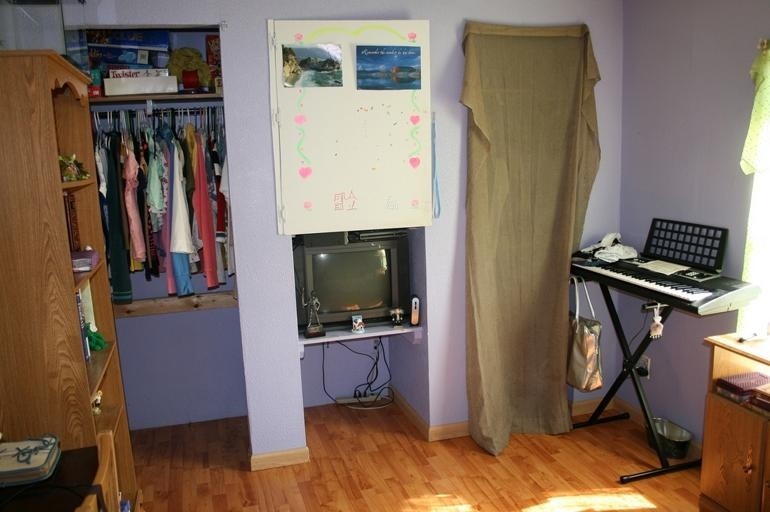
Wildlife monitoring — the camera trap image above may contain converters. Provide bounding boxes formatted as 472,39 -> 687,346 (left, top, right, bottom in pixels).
637,367 -> 648,377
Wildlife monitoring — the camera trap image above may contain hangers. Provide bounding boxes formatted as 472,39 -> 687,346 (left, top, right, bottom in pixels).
93,107 -> 224,150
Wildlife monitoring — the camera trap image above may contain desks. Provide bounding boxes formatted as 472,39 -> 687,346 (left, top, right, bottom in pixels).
299,321 -> 423,360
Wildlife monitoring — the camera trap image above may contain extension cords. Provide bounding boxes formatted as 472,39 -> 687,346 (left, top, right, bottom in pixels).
335,393 -> 382,404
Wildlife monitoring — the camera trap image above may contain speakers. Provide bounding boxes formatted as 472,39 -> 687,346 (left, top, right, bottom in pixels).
409,295 -> 420,327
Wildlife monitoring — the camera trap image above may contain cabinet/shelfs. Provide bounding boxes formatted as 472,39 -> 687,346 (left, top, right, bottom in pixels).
699,331 -> 769,512
0,50 -> 144,512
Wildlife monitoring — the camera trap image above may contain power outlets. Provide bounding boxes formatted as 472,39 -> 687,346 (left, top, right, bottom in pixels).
635,355 -> 650,379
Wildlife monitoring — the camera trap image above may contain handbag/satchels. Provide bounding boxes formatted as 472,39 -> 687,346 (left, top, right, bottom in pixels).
566,276 -> 604,393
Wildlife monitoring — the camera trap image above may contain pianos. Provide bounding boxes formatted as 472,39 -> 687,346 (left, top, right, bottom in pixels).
571,244 -> 761,319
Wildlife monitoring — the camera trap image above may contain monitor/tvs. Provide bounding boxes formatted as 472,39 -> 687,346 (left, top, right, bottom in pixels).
293,240 -> 410,325
